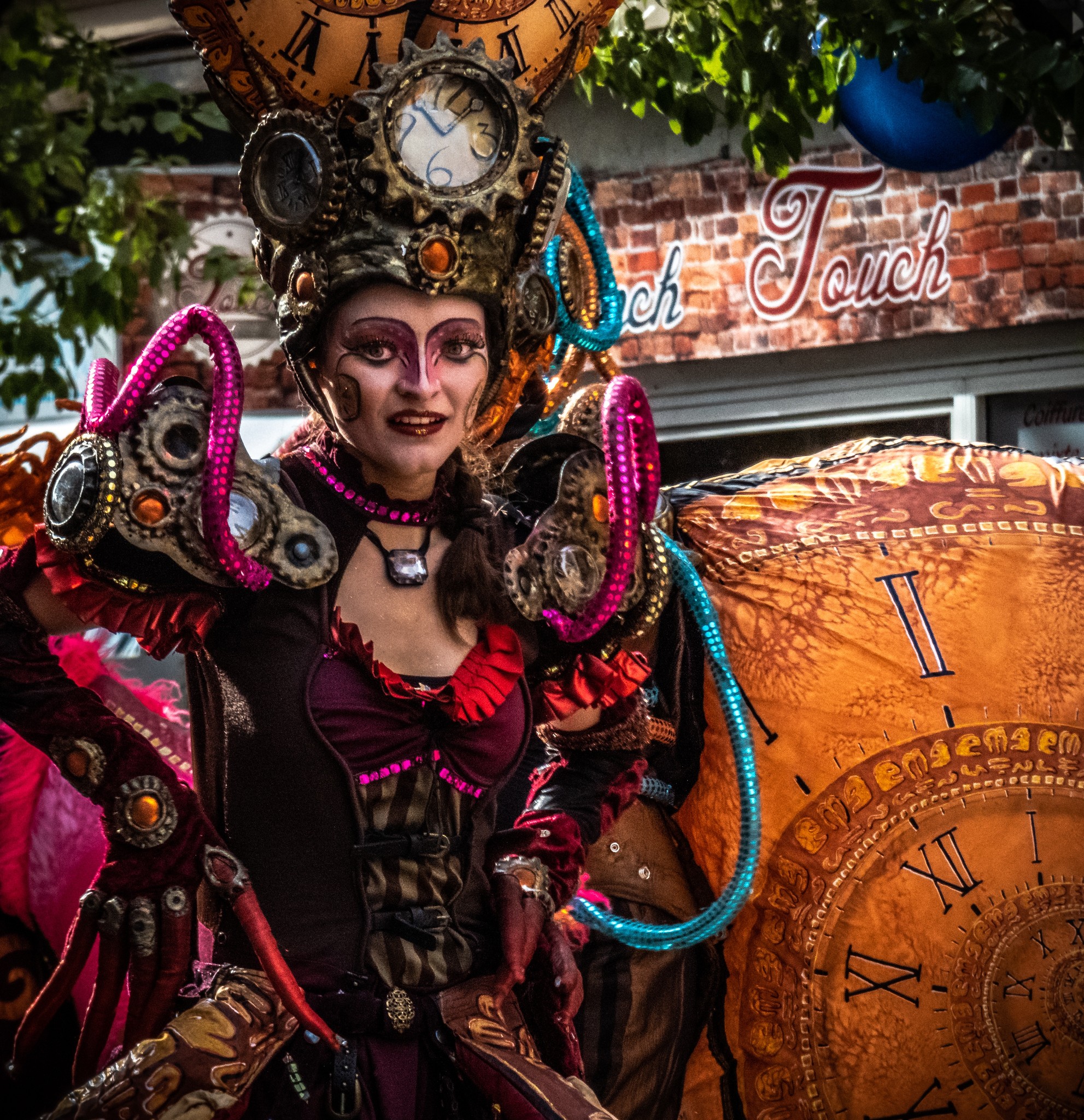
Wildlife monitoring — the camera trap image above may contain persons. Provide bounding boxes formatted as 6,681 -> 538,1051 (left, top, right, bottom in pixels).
0,33 -> 672,1120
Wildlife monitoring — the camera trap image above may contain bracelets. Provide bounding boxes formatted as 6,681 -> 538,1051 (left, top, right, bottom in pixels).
491,854 -> 557,923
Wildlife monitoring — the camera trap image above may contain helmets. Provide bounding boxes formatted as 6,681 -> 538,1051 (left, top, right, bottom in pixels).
237,29 -> 569,431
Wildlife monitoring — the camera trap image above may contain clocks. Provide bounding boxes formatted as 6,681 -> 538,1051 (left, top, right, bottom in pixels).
353,30 -> 544,228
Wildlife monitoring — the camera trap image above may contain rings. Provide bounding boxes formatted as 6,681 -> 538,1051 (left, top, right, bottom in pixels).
80,887 -> 105,924
125,896 -> 156,956
97,892 -> 129,940
202,844 -> 248,900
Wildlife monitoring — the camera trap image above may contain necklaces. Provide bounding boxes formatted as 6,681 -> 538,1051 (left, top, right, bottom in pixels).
362,522 -> 436,587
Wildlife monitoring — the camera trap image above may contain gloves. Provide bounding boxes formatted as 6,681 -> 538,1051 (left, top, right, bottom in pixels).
486,680 -> 655,1083
0,532 -> 342,1090
